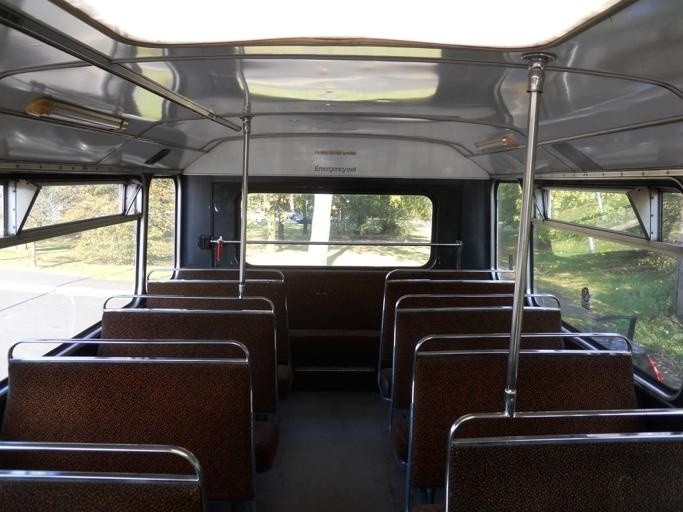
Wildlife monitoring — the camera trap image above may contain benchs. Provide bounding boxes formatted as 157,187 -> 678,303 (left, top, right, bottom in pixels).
1,269 -> 292,510
282,269 -> 395,389
377,269 -> 682,510
